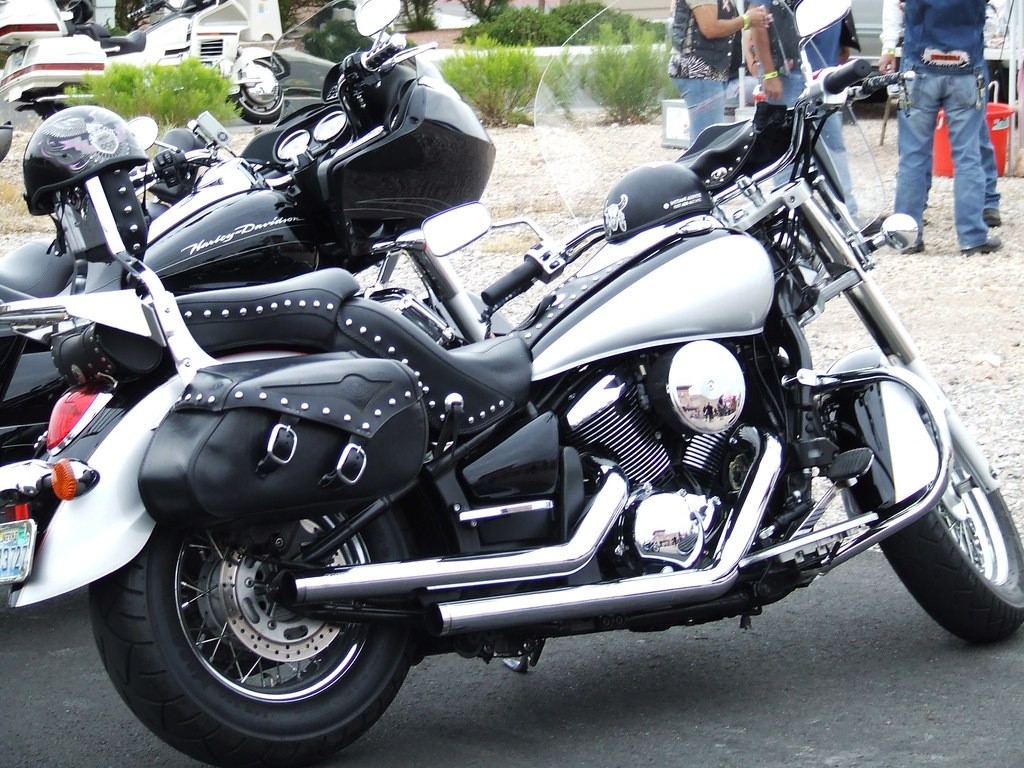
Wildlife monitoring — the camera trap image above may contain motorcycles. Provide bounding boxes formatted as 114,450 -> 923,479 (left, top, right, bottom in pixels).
0,0 -> 291,121
0,0 -> 497,522
0,0 -> 1024,768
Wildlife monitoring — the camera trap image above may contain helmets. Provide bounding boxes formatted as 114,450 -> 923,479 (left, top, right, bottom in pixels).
603,163 -> 716,244
22,105 -> 149,216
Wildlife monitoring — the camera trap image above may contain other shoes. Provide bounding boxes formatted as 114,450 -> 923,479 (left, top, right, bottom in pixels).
984,207 -> 1001,224
962,237 -> 1001,257
903,241 -> 925,254
854,215 -> 886,235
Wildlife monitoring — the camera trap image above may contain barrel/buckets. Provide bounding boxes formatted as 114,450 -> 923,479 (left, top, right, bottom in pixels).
933,103 -> 1016,178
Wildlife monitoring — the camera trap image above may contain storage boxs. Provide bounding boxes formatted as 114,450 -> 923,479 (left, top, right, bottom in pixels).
985,102 -> 1016,176
931,110 -> 954,177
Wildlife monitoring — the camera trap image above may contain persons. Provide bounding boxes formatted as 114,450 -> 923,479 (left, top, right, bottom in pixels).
667,0 -> 1006,258
671,531 -> 688,545
702,394 -> 738,423
